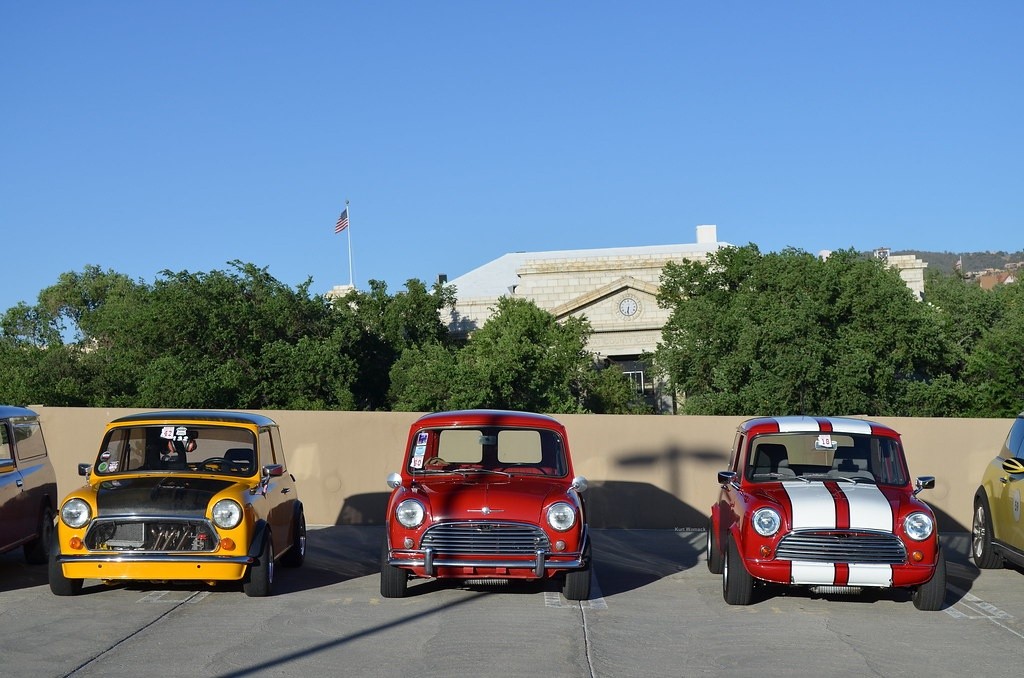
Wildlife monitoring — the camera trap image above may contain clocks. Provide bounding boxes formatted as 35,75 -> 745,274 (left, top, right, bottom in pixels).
619,298 -> 638,316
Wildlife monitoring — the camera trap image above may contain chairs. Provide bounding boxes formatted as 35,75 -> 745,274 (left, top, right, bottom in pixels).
825,446 -> 875,482
747,444 -> 796,482
220,449 -> 254,474
140,441 -> 190,470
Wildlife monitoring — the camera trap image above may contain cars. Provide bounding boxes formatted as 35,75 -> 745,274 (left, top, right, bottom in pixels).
969,410 -> 1023,575
706,413 -> 948,612
46,408 -> 306,599
0,404 -> 59,566
378,407 -> 594,603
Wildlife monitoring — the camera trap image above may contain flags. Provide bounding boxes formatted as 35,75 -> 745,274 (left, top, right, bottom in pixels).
334,208 -> 348,234
